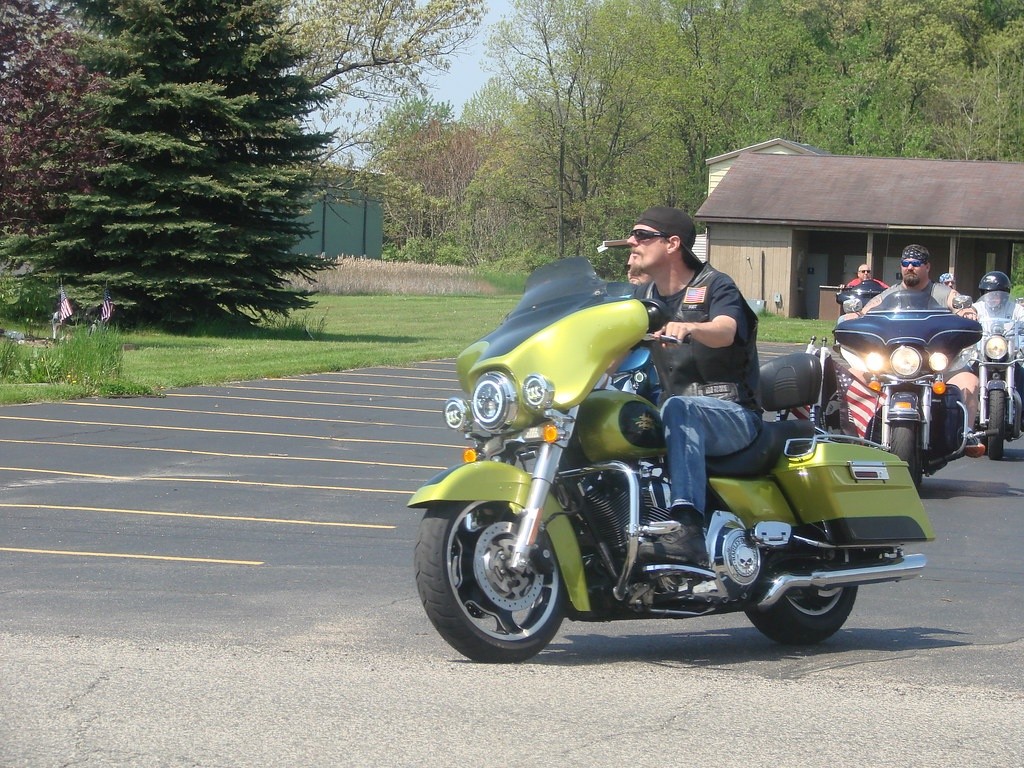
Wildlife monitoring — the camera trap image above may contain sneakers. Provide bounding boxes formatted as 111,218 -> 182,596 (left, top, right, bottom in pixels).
636,521 -> 709,569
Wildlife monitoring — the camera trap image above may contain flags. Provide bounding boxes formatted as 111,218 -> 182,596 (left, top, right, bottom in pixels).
789,349 -> 887,445
102,288 -> 113,322
60,285 -> 73,321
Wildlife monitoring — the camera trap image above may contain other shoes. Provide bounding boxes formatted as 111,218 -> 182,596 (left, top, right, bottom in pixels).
959,433 -> 985,458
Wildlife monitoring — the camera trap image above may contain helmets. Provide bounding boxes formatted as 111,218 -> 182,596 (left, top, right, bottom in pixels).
977,271 -> 1010,307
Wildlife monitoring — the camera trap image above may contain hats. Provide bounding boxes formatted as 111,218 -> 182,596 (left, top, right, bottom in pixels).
638,206 -> 704,267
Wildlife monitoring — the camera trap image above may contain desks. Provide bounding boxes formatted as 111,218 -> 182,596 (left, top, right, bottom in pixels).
819,284 -> 844,320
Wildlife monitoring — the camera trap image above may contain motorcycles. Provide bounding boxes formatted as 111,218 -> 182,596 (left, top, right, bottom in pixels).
946,292 -> 1024,459
402,259 -> 937,666
832,281 -> 887,316
835,287 -> 989,500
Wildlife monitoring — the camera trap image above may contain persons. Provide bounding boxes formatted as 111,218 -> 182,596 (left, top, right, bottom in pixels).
972,271 -> 1024,432
848,264 -> 890,288
939,271 -> 955,289
843,245 -> 985,458
626,206 -> 762,568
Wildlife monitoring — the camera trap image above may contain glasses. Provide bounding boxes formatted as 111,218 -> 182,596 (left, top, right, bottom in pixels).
629,228 -> 667,242
944,281 -> 955,285
858,269 -> 871,274
902,259 -> 924,267
